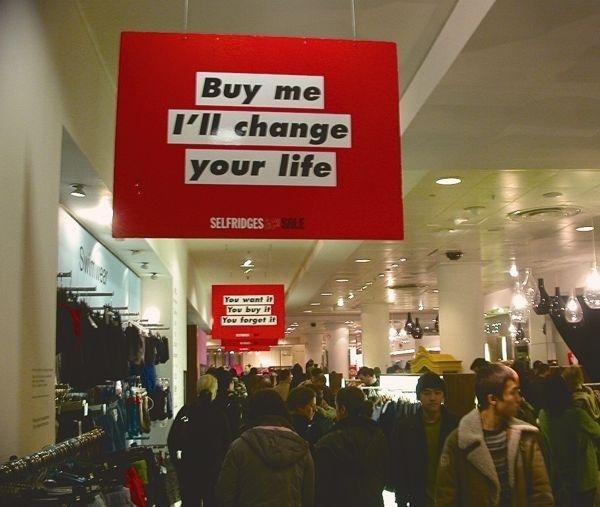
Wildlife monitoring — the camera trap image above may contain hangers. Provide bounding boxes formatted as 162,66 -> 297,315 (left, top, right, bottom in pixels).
1,429 -> 150,507
56,271 -> 170,344
53,375 -> 168,419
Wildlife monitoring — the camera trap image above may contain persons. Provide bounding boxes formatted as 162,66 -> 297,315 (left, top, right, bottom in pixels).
164,357 -> 598,506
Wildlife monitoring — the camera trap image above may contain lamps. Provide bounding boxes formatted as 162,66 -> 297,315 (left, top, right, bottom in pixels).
508,203 -> 600,324
68,182 -> 91,202
389,311 -> 423,347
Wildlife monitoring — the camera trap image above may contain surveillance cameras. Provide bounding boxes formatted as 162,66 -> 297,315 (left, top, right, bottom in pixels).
445,251 -> 464,261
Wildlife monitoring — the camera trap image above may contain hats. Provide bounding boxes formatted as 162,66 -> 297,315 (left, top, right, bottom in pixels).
416,371 -> 448,401
